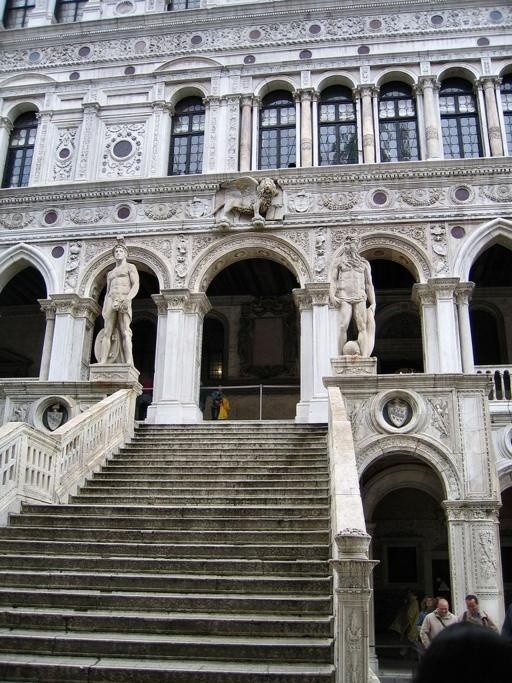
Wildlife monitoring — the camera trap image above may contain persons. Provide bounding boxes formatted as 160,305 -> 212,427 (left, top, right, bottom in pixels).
331,235 -> 376,357
398,591 -> 419,654
414,596 -> 436,647
139,379 -> 153,419
457,595 -> 499,631
418,596 -> 459,649
500,604 -> 511,637
417,621 -> 511,682
96,244 -> 141,364
210,384 -> 224,419
